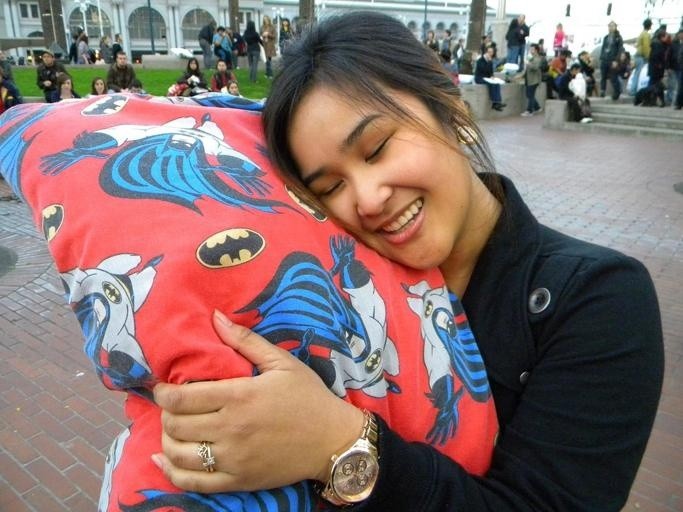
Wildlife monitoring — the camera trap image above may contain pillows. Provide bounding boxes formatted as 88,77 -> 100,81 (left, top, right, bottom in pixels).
0,91 -> 500,512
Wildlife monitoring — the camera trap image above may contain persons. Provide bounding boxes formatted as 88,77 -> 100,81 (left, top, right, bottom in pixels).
195,12 -> 300,84
424,13 -> 683,129
1,26 -> 239,112
148,12 -> 663,511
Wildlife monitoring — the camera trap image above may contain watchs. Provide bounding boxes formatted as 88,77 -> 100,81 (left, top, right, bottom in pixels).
313,402 -> 383,507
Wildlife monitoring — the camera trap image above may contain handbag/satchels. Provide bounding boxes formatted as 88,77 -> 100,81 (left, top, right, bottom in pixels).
540,57 -> 550,74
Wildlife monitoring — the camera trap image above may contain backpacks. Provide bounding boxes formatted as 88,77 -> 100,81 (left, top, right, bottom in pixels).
617,50 -> 633,81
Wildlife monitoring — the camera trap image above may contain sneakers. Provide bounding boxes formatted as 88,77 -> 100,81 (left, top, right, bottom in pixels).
520,110 -> 533,117
612,87 -> 622,100
580,117 -> 594,124
491,103 -> 507,112
533,107 -> 543,114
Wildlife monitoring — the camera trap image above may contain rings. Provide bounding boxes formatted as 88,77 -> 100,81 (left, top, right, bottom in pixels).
198,442 -> 215,473
